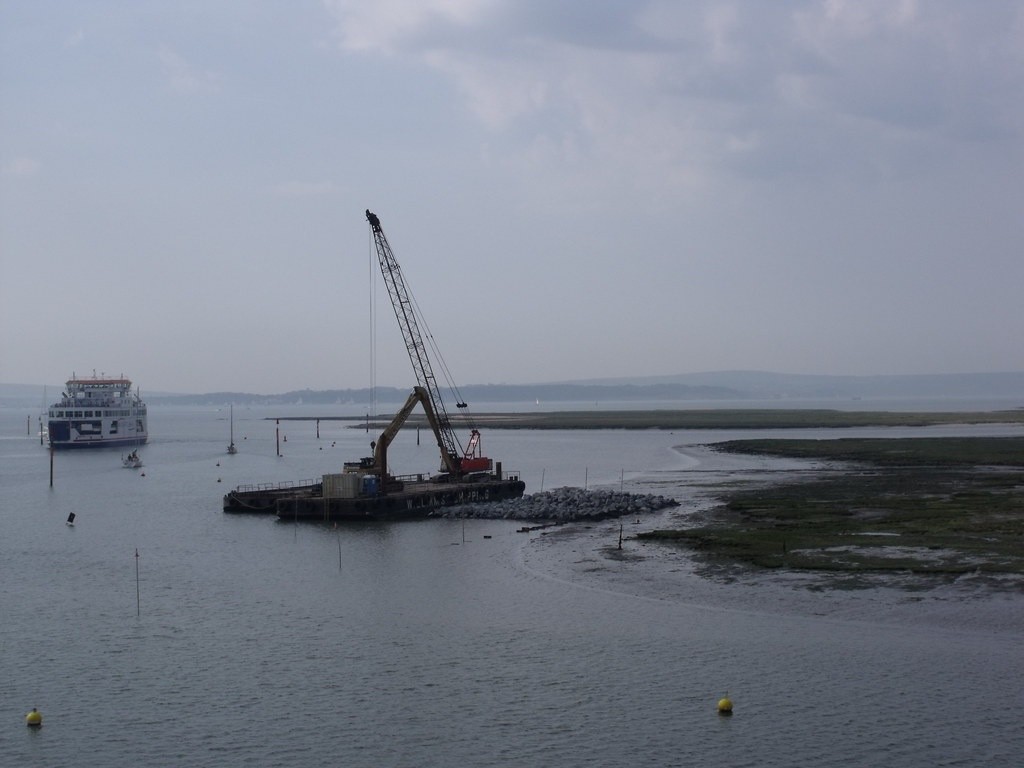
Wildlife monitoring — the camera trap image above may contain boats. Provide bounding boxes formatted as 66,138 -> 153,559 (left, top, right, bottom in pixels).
47,368 -> 149,450
224,467 -> 432,514
122,455 -> 144,469
274,464 -> 526,518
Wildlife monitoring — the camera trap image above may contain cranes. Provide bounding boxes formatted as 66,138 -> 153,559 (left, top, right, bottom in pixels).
363,208 -> 498,483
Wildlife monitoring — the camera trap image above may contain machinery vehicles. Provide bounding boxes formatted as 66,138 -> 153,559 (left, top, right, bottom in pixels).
347,385 -> 469,492
